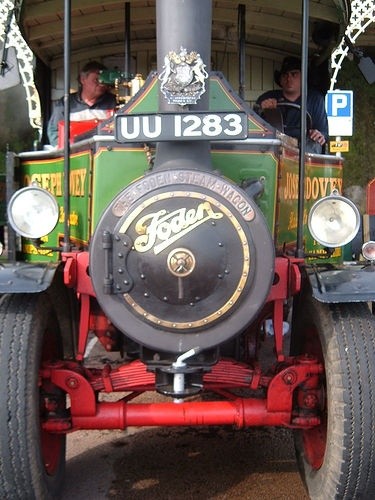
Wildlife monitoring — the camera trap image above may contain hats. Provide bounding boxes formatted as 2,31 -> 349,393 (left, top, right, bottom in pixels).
273,56 -> 301,89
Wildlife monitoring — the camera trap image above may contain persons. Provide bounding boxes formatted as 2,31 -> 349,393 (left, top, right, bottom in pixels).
253,56 -> 329,154
47,60 -> 125,149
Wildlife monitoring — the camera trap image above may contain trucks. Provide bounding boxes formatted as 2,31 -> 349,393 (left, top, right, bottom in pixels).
0,0 -> 375,499
